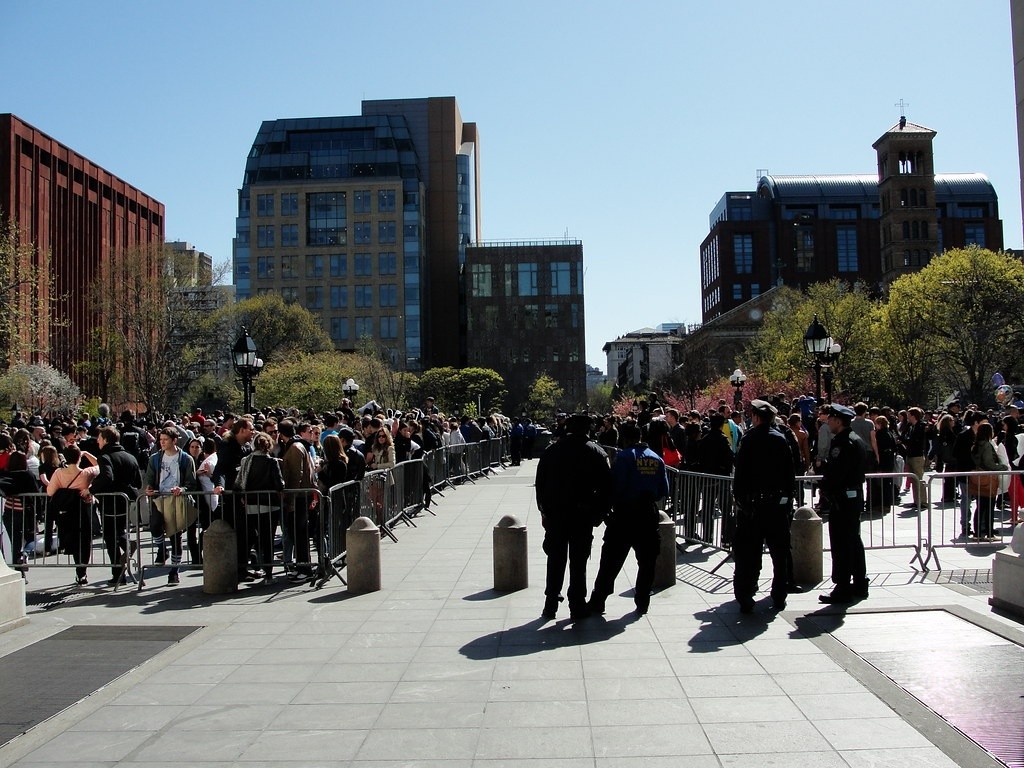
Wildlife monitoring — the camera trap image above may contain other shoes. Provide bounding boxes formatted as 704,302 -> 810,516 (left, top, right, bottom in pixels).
120,541 -> 137,563
509,461 -> 516,467
992,529 -> 999,534
515,462 -> 520,466
908,504 -> 917,507
819,588 -> 867,603
107,579 -> 128,585
544,609 -> 555,619
242,576 -> 255,581
921,505 -> 927,508
76,577 -> 88,584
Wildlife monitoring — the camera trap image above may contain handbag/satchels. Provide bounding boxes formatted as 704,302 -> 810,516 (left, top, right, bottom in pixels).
969,469 -> 999,497
662,435 -> 683,465
0,448 -> 15,469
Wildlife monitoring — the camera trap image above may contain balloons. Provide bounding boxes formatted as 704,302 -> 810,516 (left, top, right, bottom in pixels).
991,373 -> 1012,408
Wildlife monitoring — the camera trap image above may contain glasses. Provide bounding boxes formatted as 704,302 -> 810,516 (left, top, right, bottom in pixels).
267,430 -> 279,435
377,435 -> 387,438
203,424 -> 213,428
819,413 -> 824,415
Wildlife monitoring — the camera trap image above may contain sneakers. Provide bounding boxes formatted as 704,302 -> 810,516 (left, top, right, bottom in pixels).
154,554 -> 169,565
168,571 -> 179,586
288,573 -> 309,582
288,570 -> 299,579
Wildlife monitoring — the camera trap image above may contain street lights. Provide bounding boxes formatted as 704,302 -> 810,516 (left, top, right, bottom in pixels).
730,369 -> 747,402
238,352 -> 263,411
821,336 -> 841,405
342,378 -> 359,402
231,325 -> 257,414
801,314 -> 832,405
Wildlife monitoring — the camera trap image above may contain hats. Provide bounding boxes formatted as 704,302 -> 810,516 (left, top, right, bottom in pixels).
827,404 -> 855,420
750,399 -> 778,417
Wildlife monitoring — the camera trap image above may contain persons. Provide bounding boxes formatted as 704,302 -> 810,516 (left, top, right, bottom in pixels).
733,399 -> 795,612
0,392 -> 1024,584
585,425 -> 670,615
819,403 -> 869,601
536,415 -> 610,621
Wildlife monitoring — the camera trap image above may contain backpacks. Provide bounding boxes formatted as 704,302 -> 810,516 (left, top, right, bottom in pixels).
49,471 -> 82,526
